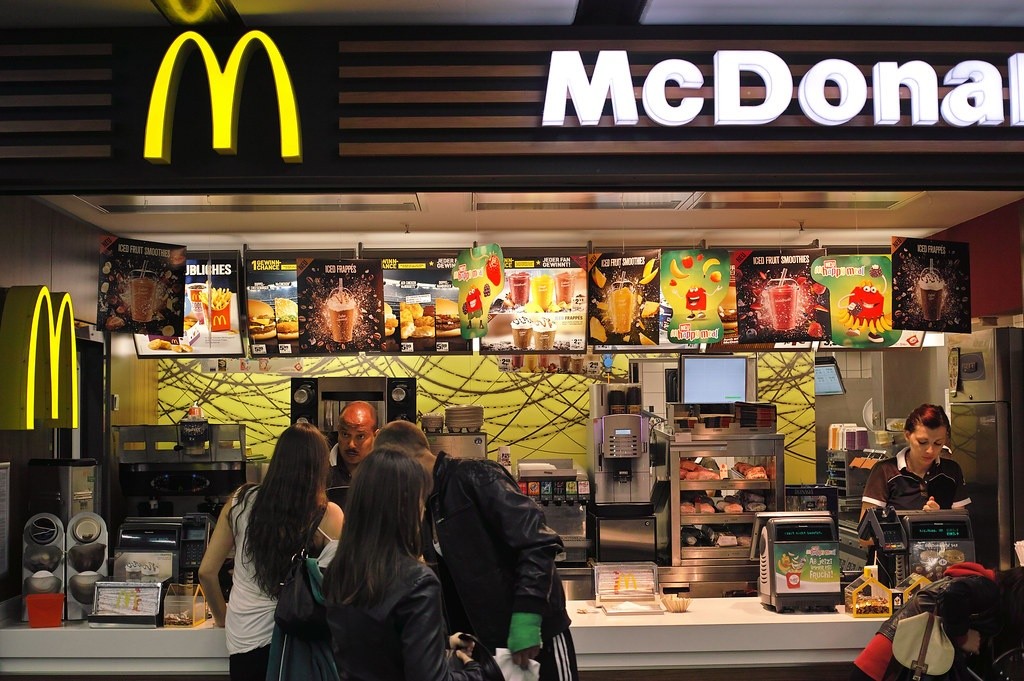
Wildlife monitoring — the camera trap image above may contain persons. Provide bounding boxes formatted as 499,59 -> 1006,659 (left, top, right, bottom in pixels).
196,396 -> 579,681
858,404 -> 971,549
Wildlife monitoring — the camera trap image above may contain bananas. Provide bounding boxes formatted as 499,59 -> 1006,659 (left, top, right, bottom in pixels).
670,259 -> 689,279
703,258 -> 720,278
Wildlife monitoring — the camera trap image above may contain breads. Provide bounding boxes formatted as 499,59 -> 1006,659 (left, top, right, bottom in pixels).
680,458 -> 770,547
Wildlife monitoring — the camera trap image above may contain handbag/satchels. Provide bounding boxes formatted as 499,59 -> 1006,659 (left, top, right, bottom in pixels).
885,591 -> 969,681
274,501 -> 331,640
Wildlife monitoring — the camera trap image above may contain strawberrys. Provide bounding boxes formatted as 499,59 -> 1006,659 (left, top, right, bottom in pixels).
735,269 -> 830,337
485,251 -> 501,285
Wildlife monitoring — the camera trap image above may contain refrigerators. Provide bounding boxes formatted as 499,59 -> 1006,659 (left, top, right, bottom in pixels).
948,326 -> 1024,571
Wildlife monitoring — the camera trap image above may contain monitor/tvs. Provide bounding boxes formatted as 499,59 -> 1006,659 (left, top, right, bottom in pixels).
815,364 -> 846,396
682,355 -> 746,415
750,510 -> 831,562
895,508 -> 971,520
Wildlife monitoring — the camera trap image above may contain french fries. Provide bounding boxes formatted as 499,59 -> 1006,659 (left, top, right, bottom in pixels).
198,280 -> 233,310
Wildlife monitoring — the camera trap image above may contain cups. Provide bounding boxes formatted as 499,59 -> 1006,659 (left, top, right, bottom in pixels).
130,270 -> 158,322
151,500 -> 158,508
508,272 -> 531,306
497,446 -> 511,477
556,272 -> 576,305
920,268 -> 944,321
327,287 -> 355,342
766,278 -> 799,330
611,280 -> 636,333
529,272 -> 553,311
188,283 -> 210,324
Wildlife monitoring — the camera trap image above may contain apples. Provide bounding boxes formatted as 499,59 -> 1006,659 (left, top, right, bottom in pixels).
682,256 -> 694,269
710,271 -> 722,282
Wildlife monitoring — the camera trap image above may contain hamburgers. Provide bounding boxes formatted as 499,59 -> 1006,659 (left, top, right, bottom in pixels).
247,298 -> 276,340
275,298 -> 300,340
383,298 -> 462,337
920,549 -> 966,568
